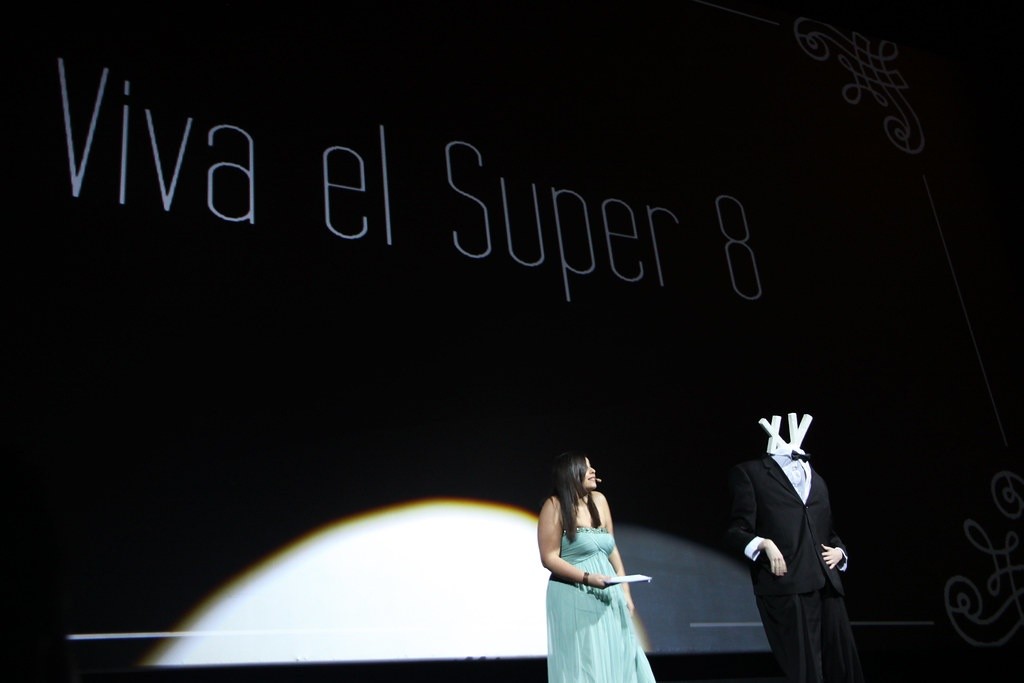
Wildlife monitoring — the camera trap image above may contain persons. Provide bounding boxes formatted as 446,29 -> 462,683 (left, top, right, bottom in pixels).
729,443 -> 865,682
537,451 -> 657,683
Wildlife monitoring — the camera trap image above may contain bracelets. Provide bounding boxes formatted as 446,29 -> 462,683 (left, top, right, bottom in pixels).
582,571 -> 589,588
